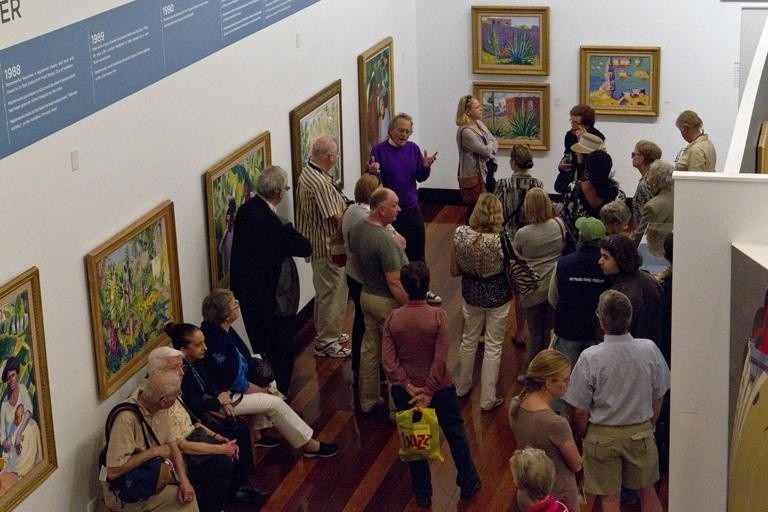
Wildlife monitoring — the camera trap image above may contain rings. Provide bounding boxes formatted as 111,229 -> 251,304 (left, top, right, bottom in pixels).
188,497 -> 192,499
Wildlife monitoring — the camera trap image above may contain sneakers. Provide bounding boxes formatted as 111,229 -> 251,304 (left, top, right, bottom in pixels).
334,330 -> 352,339
302,442 -> 340,460
428,291 -> 443,303
234,484 -> 269,504
255,437 -> 281,449
512,335 -> 527,352
314,341 -> 353,359
483,396 -> 505,413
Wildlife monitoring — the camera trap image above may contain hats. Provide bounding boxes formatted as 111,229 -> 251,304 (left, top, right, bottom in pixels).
575,216 -> 607,241
570,132 -> 604,154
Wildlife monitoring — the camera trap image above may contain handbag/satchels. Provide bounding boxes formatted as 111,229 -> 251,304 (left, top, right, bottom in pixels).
182,451 -> 215,490
457,126 -> 488,206
244,356 -> 278,390
97,401 -> 174,504
498,231 -> 539,298
554,146 -> 579,195
396,406 -> 445,464
328,206 -> 352,267
192,389 -> 240,428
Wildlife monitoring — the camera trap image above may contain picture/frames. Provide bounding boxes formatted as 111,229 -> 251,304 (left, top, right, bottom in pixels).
472,81 -> 551,152
358,36 -> 397,176
205,130 -> 272,292
0,266 -> 59,512
471,5 -> 551,76
87,199 -> 184,401
578,45 -> 661,117
289,80 -> 344,196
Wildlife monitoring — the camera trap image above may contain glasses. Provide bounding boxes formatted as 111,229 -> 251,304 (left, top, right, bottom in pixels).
173,362 -> 184,372
231,300 -> 240,312
282,186 -> 293,191
631,150 -> 640,157
594,309 -> 601,318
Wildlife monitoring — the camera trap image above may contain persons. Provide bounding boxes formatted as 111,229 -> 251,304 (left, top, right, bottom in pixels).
129,346 -> 268,511
450,192 -> 523,412
565,133 -> 614,186
562,150 -> 626,224
456,96 -> 498,212
14,404 -> 25,426
634,160 -> 674,255
493,144 -> 543,348
294,136 -> 352,358
513,187 -> 571,385
218,201 -> 235,277
556,103 -> 606,179
631,140 -> 662,236
341,175 -> 407,385
380,261 -> 483,508
100,372 -> 199,512
229,166 -> 317,402
656,233 -> 673,432
346,188 -> 412,427
594,235 -> 665,353
377,97 -> 390,144
160,320 -> 255,481
546,218 -> 608,415
508,349 -> 584,512
241,182 -> 255,203
201,290 -> 341,459
674,111 -> 716,172
559,290 -> 672,512
363,112 -> 442,304
509,447 -> 576,512
595,199 -> 630,237
0,357 -> 33,475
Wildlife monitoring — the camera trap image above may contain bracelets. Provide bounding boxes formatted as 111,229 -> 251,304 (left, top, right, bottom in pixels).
213,433 -> 218,438
